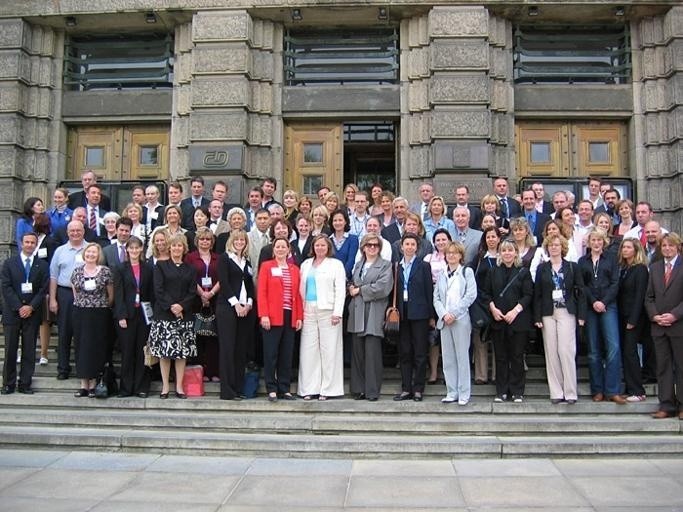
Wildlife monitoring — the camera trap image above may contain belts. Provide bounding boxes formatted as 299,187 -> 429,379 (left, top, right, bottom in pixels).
57,284 -> 73,291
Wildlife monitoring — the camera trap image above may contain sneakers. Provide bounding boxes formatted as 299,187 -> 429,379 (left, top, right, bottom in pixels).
621,394 -> 630,398
39,358 -> 49,365
511,393 -> 524,402
626,395 -> 646,402
493,394 -> 507,403
16,356 -> 22,363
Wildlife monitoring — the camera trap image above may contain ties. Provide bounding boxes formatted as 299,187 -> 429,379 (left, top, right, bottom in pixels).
423,204 -> 428,221
25,259 -> 31,281
195,202 -> 198,209
120,246 -> 125,264
262,234 -> 267,246
500,199 -> 507,217
639,229 -> 646,249
664,262 -> 672,283
90,209 -> 97,231
528,215 -> 534,232
460,232 -> 465,245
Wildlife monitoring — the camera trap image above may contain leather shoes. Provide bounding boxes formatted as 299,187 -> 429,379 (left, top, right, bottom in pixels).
74,389 -> 89,397
232,396 -> 243,401
176,391 -> 187,399
278,392 -> 295,399
567,400 -> 575,404
592,392 -> 604,402
458,398 -> 469,405
238,394 -> 248,399
18,387 -> 33,394
57,372 -> 69,380
160,391 -> 169,399
677,410 -> 682,420
492,380 -> 497,386
551,399 -> 563,404
137,391 -> 147,397
475,379 -> 487,385
88,389 -> 96,398
1,384 -> 15,394
651,410 -> 677,419
268,393 -> 278,401
441,395 -> 457,402
118,393 -> 133,397
412,392 -> 423,401
393,393 -> 413,401
76,371 -> 81,378
608,394 -> 626,404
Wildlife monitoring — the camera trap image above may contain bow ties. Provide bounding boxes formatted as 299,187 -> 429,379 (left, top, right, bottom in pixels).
210,220 -> 216,224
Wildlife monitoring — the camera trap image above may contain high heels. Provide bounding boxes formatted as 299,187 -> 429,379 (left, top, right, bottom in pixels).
353,392 -> 366,400
428,377 -> 438,385
303,395 -> 318,400
444,379 -> 446,385
369,397 -> 377,401
318,395 -> 329,401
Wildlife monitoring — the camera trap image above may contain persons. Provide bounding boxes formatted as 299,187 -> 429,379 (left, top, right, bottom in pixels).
617,237 -> 648,401
587,178 -> 602,210
146,234 -> 196,398
296,196 -> 311,216
215,229 -> 254,400
247,186 -> 264,232
368,184 -> 385,226
372,191 -> 396,230
554,208 -> 583,258
391,214 -> 432,369
446,185 -> 484,230
512,194 -> 523,212
433,241 -> 477,406
492,179 -> 522,220
152,183 -> 182,232
393,234 -> 433,401
348,191 -> 372,246
316,188 -> 345,210
289,213 -> 316,268
351,217 -> 392,275
612,200 -> 638,236
297,234 -> 345,400
70,244 -> 113,397
132,186 -> 147,225
409,185 -> 434,213
641,222 -> 662,385
267,204 -> 296,242
15,198 -> 55,256
591,181 -> 613,222
470,226 -> 502,384
206,199 -> 225,253
146,228 -> 170,383
92,211 -> 120,250
180,176 -> 211,229
603,189 -> 620,225
111,237 -> 152,398
53,207 -> 97,245
339,184 -> 358,216
564,190 -> 575,215
184,206 -> 217,254
246,209 -> 271,368
421,197 -> 457,245
44,188 -> 73,245
509,217 -> 537,349
381,198 -> 425,248
48,221 -> 93,380
577,227 -> 626,403
184,226 -> 220,382
122,203 -> 151,244
200,180 -> 232,221
644,233 -> 682,419
67,172 -> 110,213
423,229 -> 452,385
82,185 -> 110,248
327,209 -> 358,368
481,238 -> 533,402
243,178 -> 276,208
586,213 -> 623,254
346,232 -> 393,400
322,192 -> 339,216
481,195 -> 510,241
0,232 -> 49,394
100,217 -> 132,355
256,219 -> 300,279
309,206 -> 332,240
530,183 -> 555,215
447,207 -> 483,267
481,215 -> 496,232
530,220 -> 577,282
623,202 -> 669,240
257,238 -> 303,401
549,191 -> 567,220
282,190 -> 299,226
140,185 -> 166,243
576,200 -> 593,235
532,235 -> 586,403
145,205 -> 188,262
15,213 -> 53,365
520,189 -> 552,247
212,208 -> 246,254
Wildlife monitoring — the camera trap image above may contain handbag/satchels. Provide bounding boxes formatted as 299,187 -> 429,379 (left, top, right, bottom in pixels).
143,341 -> 160,367
428,326 -> 440,347
95,360 -> 120,398
462,266 -> 488,329
493,267 -> 525,303
240,362 -> 261,398
193,303 -> 218,336
475,257 -> 482,277
480,316 -> 514,344
384,261 -> 399,335
176,365 -> 204,397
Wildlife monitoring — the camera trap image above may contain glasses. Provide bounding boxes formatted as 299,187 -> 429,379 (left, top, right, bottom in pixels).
200,237 -> 210,241
355,200 -> 366,204
364,244 -> 380,248
